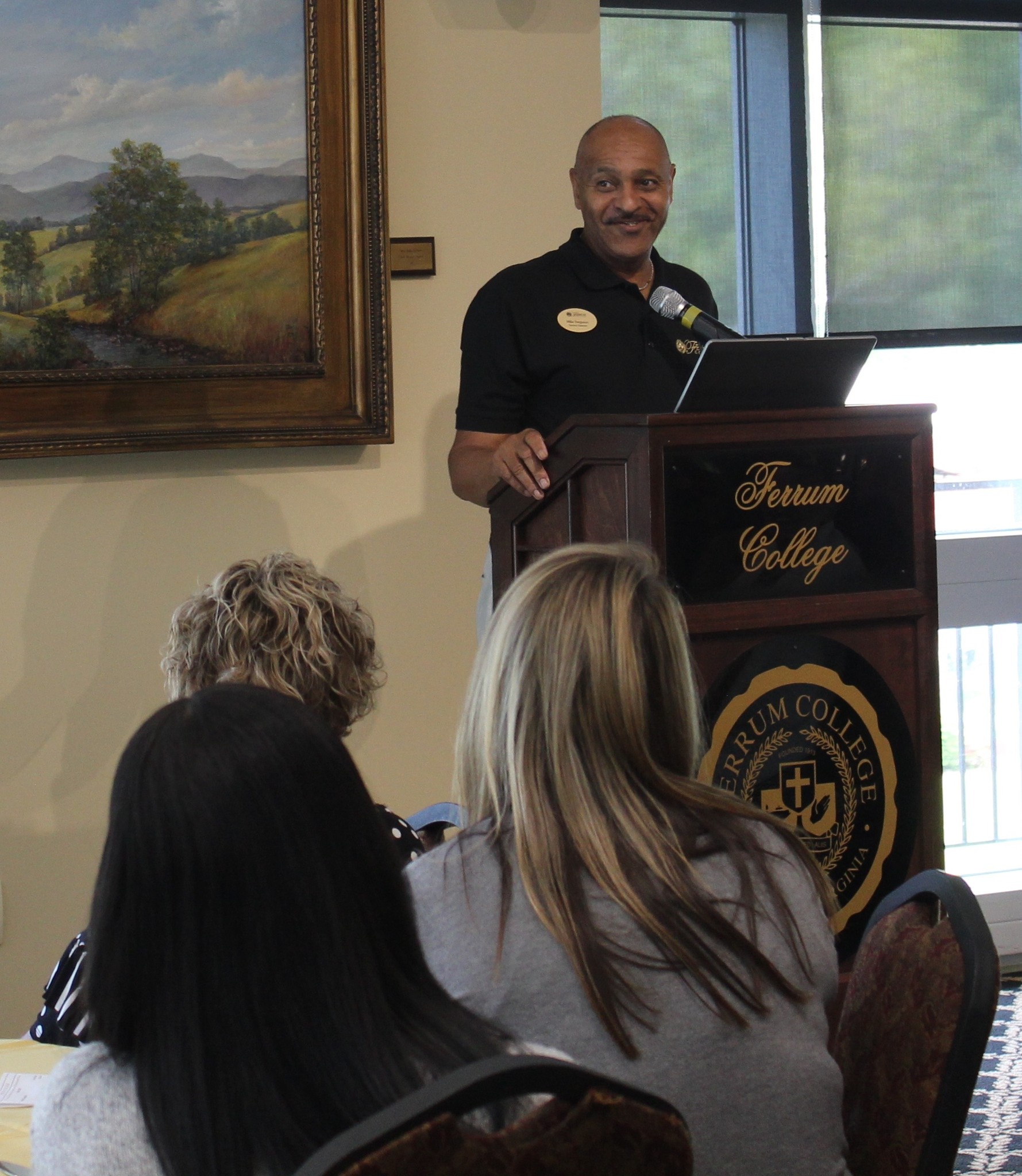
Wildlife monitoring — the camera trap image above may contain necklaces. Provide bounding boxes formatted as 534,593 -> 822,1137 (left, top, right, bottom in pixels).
638,258 -> 654,290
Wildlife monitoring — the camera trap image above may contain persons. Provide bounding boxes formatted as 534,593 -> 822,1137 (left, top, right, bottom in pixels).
405,542 -> 854,1176
23,554 -> 578,1176
448,114 -> 719,642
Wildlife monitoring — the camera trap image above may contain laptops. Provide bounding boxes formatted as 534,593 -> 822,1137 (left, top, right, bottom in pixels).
673,335 -> 878,413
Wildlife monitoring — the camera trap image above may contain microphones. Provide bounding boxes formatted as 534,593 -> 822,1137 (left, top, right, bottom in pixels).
649,285 -> 747,341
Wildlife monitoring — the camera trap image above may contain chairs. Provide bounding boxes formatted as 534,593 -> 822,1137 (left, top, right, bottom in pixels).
302,1052 -> 693,1176
831,868 -> 1000,1176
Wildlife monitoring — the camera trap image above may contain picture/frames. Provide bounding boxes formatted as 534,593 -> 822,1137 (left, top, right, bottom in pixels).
0,0 -> 394,459
